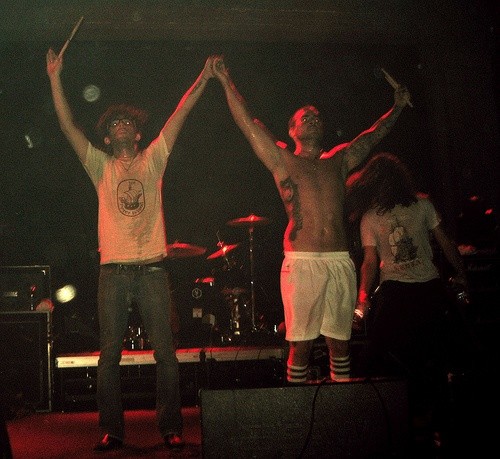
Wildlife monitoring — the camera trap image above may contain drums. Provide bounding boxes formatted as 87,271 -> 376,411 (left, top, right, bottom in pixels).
223,284 -> 267,334
165,282 -> 237,347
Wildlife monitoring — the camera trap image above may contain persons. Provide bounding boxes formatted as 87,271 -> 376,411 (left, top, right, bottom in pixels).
348,152 -> 471,450
212,60 -> 411,384
47,47 -> 223,452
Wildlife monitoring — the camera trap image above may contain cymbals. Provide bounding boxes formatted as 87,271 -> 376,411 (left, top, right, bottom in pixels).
164,243 -> 206,257
224,215 -> 274,225
205,242 -> 243,260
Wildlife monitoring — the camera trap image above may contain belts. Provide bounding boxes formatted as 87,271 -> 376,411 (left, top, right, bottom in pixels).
105,262 -> 169,273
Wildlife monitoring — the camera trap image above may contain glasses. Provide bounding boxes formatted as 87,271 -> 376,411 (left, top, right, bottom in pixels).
108,118 -> 134,130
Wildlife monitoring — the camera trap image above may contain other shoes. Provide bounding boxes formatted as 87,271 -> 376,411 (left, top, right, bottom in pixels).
164,434 -> 184,452
95,435 -> 123,451
